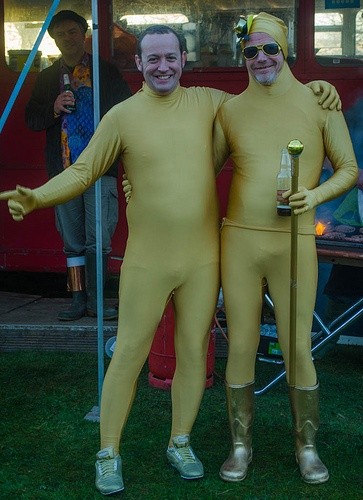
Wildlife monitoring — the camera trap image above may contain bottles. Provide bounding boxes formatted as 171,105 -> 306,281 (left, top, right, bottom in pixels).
236,36 -> 245,67
276,148 -> 292,216
63,72 -> 76,112
217,43 -> 234,67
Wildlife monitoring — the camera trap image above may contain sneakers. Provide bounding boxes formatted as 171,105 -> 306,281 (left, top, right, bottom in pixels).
94,446 -> 125,495
166,435 -> 205,480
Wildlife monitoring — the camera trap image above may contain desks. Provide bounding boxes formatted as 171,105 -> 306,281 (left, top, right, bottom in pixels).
254,231 -> 363,394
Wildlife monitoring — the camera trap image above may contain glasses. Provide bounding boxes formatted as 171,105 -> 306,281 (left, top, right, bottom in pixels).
241,42 -> 281,61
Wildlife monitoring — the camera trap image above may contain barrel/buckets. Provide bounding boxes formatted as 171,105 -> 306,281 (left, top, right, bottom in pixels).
7,50 -> 42,73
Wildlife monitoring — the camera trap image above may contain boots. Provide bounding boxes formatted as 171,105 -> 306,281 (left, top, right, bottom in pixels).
286,382 -> 330,485
85,252 -> 118,320
218,381 -> 256,483
58,255 -> 85,320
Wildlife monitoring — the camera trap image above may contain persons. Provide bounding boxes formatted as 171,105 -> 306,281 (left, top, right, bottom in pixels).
0,25 -> 342,494
25,10 -> 132,320
122,13 -> 358,485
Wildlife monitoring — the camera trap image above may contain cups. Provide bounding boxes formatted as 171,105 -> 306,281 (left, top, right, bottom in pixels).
105,336 -> 117,358
